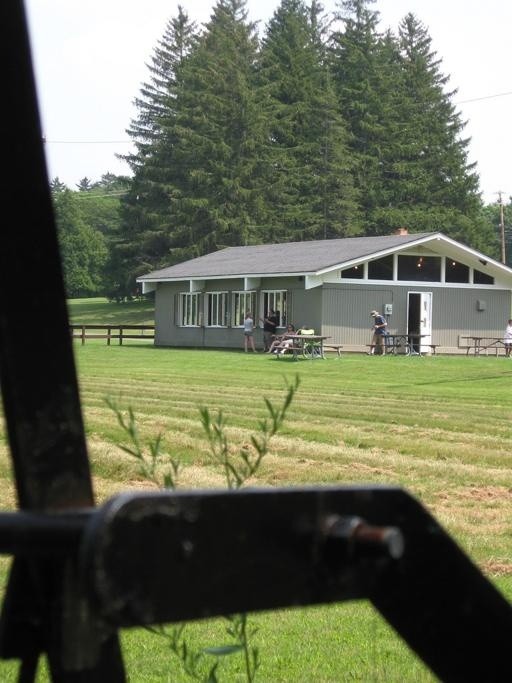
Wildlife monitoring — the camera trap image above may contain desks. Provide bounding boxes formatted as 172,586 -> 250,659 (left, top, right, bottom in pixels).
282,335 -> 512,356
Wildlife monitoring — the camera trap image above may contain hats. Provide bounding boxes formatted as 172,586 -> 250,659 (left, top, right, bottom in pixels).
370,311 -> 378,317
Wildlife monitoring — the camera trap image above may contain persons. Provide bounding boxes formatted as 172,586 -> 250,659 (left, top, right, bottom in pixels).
267,323 -> 308,355
243,312 -> 258,353
503,320 -> 512,357
367,310 -> 388,356
260,308 -> 276,353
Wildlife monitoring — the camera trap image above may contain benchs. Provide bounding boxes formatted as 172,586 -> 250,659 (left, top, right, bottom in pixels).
273,346 -> 302,350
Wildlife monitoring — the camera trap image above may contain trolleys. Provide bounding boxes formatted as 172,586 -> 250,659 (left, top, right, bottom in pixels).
295,328 -> 320,359
